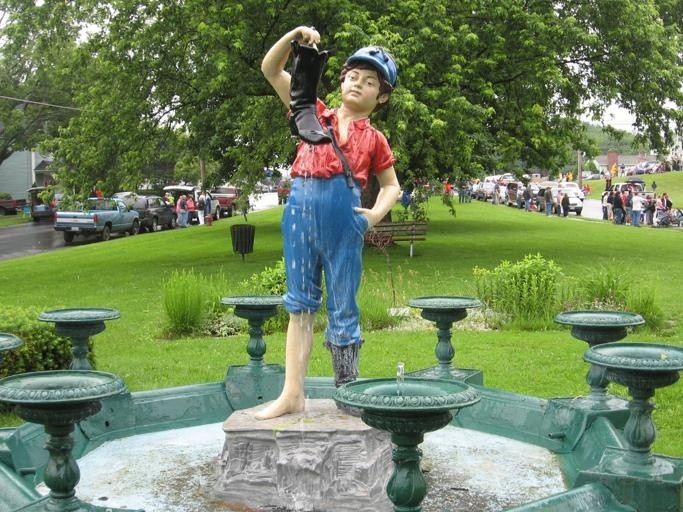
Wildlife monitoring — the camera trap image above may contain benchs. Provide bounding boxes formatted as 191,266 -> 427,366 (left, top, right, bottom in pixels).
367,220 -> 427,258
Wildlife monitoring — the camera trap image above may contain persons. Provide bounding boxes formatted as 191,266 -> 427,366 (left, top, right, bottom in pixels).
254,24 -> 400,422
439,163 -> 672,229
163,190 -> 212,227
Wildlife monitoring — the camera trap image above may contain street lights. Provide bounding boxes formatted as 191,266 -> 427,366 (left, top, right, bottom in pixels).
578,150 -> 586,191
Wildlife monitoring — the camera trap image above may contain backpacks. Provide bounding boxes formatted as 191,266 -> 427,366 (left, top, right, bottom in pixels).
198,199 -> 204,210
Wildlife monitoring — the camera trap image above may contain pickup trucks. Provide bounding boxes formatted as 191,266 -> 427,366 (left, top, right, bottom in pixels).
53,197 -> 141,241
601,182 -> 655,212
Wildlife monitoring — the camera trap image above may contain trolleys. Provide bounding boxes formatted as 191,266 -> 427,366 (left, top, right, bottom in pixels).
0,198 -> 32,216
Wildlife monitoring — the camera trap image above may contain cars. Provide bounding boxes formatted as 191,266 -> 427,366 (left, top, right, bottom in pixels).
581,170 -> 592,177
111,182 -> 249,231
587,174 -> 600,180
625,160 -> 658,175
452,173 -> 585,216
253,176 -> 293,201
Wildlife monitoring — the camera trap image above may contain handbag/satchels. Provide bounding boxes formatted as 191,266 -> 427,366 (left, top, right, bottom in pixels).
528,203 -> 537,210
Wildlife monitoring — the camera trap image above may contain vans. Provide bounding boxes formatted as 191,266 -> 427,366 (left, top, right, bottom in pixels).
26,185 -> 66,221
